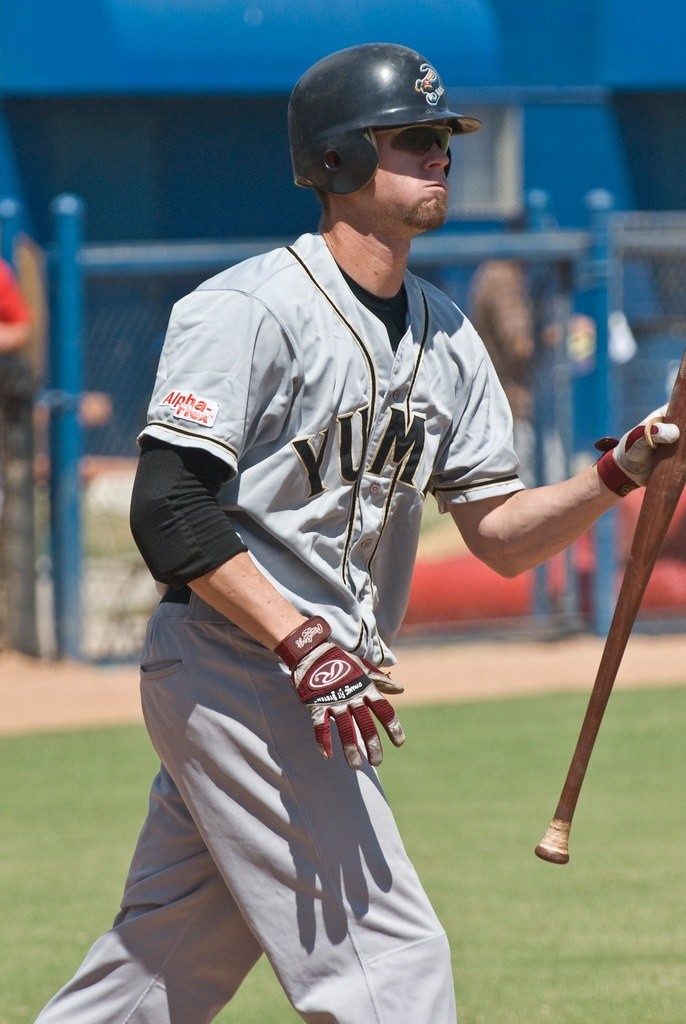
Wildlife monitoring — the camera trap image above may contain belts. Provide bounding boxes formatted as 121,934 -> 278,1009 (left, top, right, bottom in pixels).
159,586 -> 192,605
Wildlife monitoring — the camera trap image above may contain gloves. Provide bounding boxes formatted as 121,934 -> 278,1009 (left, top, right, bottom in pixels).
595,403 -> 680,497
274,616 -> 406,770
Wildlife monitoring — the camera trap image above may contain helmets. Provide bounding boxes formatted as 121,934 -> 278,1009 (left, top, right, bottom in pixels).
287,43 -> 483,194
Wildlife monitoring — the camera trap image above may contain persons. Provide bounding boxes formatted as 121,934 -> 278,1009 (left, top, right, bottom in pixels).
33,42 -> 678,1023
469,209 -> 594,502
0,261 -> 31,461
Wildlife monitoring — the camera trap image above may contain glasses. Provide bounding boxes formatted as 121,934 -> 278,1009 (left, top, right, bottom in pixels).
374,123 -> 453,156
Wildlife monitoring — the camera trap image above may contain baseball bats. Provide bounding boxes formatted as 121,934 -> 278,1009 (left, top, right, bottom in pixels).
532,350 -> 685,866
